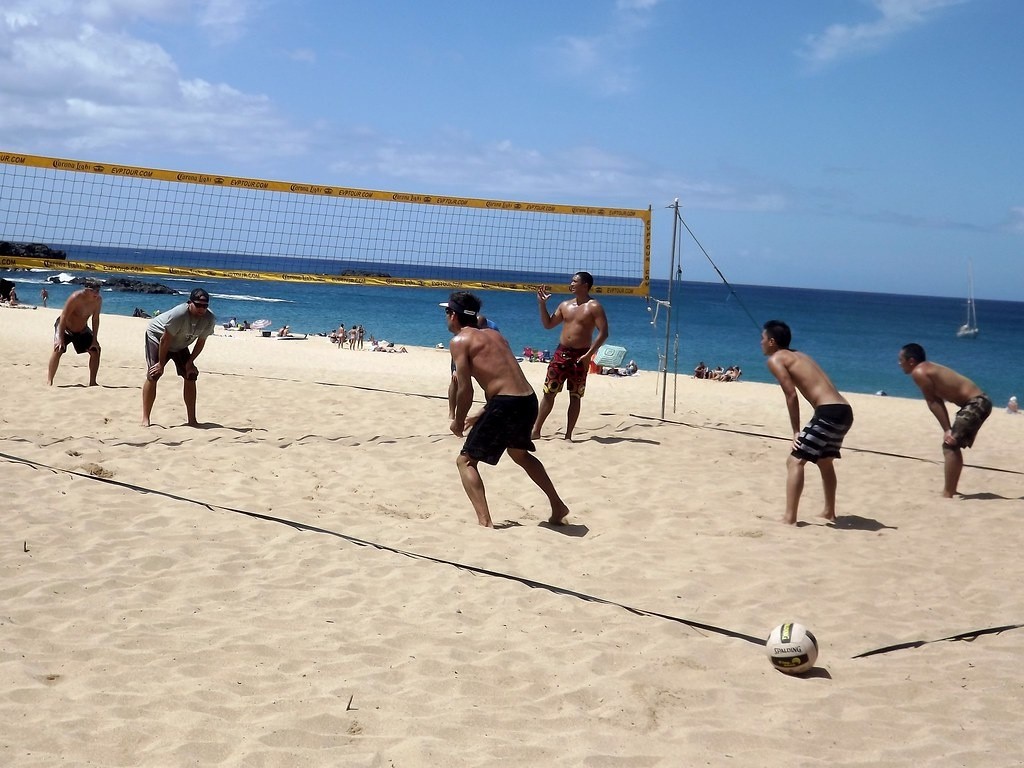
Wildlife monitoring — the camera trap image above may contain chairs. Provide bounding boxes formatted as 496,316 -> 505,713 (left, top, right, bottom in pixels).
705,367 -> 742,382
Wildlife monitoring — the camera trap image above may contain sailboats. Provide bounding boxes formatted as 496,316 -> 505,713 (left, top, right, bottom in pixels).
956,261 -> 980,340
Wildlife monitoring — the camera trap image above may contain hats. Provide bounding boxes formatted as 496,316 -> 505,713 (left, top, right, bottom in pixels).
286,325 -> 290,329
438,299 -> 477,315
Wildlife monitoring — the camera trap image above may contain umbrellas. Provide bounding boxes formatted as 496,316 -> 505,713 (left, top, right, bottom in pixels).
593,344 -> 626,367
251,319 -> 271,331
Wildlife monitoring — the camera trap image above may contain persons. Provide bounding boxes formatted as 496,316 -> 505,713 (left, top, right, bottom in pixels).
278,326 -> 293,337
140,288 -> 216,427
898,344 -> 992,499
761,320 -> 854,527
369,340 -> 408,353
628,360 -> 638,374
230,317 -> 237,327
449,315 -> 500,420
331,329 -> 338,342
357,325 -> 366,349
244,321 -> 250,329
439,291 -> 570,528
531,272 -> 609,443
348,325 -> 358,350
133,307 -> 161,319
47,277 -> 102,386
9,287 -> 19,306
694,362 -> 742,382
41,287 -> 49,307
338,324 -> 344,348
343,328 -> 348,343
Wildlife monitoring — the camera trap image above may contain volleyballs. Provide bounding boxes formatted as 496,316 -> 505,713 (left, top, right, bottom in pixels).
765,623 -> 819,674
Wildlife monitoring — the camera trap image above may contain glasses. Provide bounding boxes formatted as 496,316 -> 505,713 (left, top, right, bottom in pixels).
85,288 -> 100,293
191,299 -> 209,309
